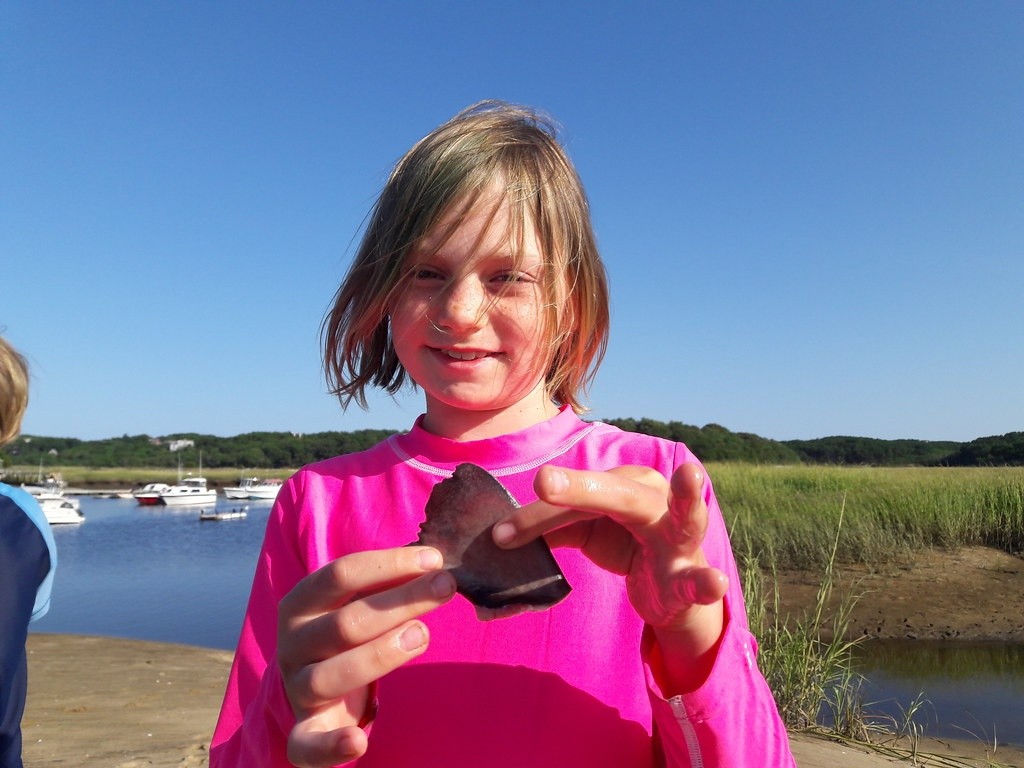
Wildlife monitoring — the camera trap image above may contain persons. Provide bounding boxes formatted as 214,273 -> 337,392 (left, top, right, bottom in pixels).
206,96 -> 796,768
0,337 -> 59,768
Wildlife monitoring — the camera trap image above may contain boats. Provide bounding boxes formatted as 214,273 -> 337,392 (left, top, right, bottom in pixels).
133,452 -> 180,504
159,450 -> 217,505
34,492 -> 85,524
199,506 -> 248,520
20,483 -> 64,494
223,477 -> 282,498
